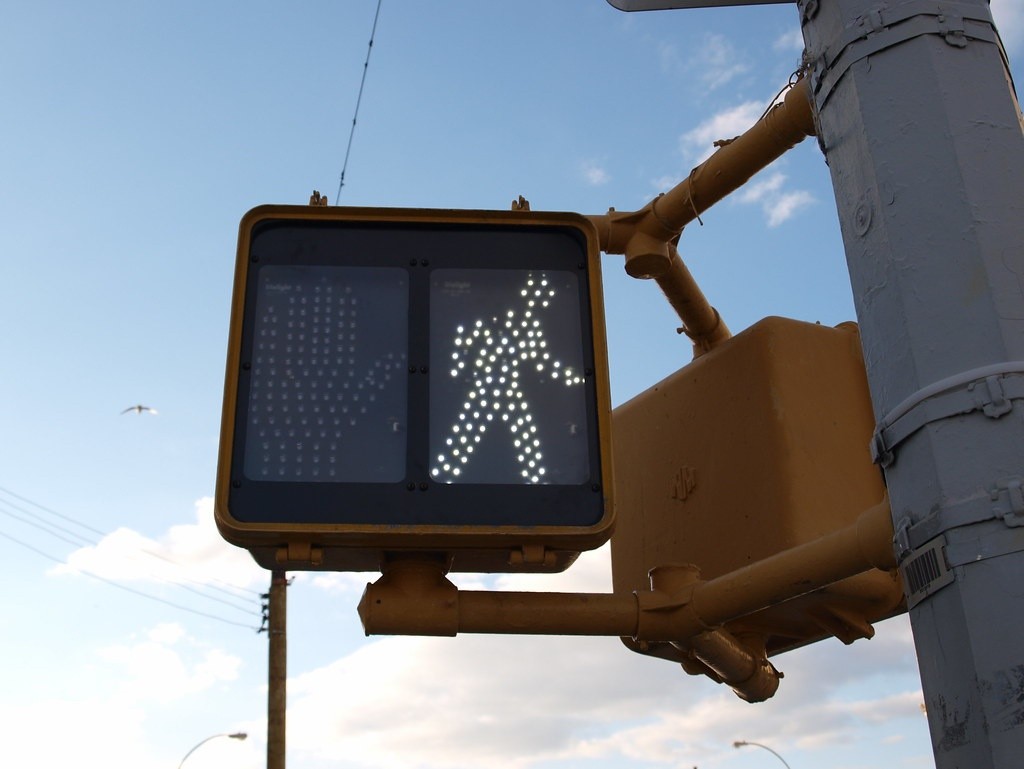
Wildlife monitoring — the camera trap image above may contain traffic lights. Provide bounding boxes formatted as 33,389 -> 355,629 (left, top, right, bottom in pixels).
212,189 -> 621,576
